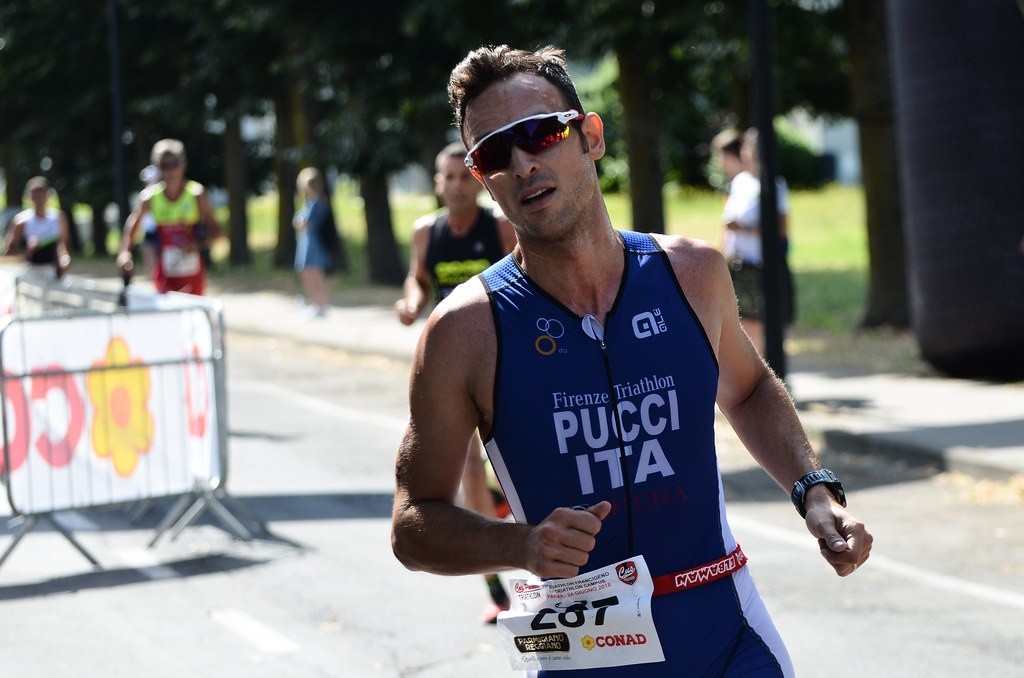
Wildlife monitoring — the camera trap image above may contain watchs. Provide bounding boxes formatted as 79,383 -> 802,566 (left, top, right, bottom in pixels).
791,469 -> 847,519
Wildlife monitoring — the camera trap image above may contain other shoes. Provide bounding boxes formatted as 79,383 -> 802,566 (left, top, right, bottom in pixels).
485,599 -> 510,623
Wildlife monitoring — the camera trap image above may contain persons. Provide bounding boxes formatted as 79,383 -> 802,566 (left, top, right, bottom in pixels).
394,142 -> 518,626
292,168 -> 331,319
389,45 -> 872,678
116,138 -> 223,292
3,177 -> 71,280
712,127 -> 796,366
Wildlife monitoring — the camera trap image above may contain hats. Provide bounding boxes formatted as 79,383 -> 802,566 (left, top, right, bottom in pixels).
149,138 -> 184,167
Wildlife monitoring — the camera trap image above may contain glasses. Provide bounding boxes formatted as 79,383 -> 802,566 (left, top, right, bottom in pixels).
464,109 -> 586,177
